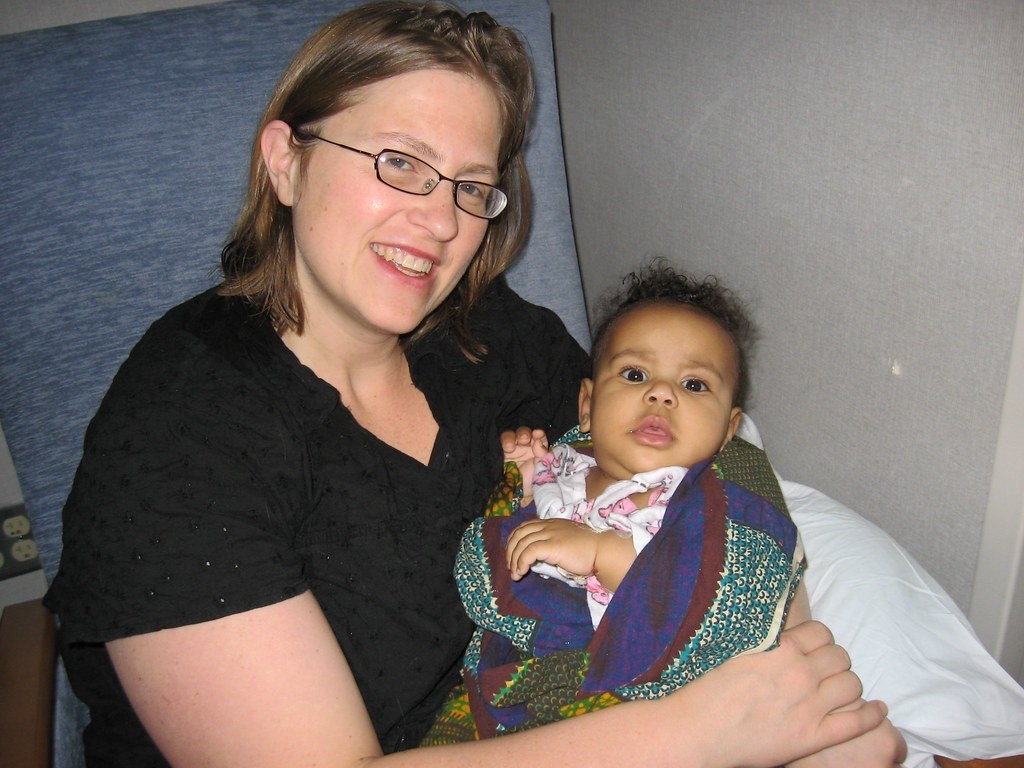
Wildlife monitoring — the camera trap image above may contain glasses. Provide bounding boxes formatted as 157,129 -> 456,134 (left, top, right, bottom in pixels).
292,126 -> 510,222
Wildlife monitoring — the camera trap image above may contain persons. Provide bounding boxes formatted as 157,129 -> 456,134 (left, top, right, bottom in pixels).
41,0 -> 907,768
421,255 -> 808,748
